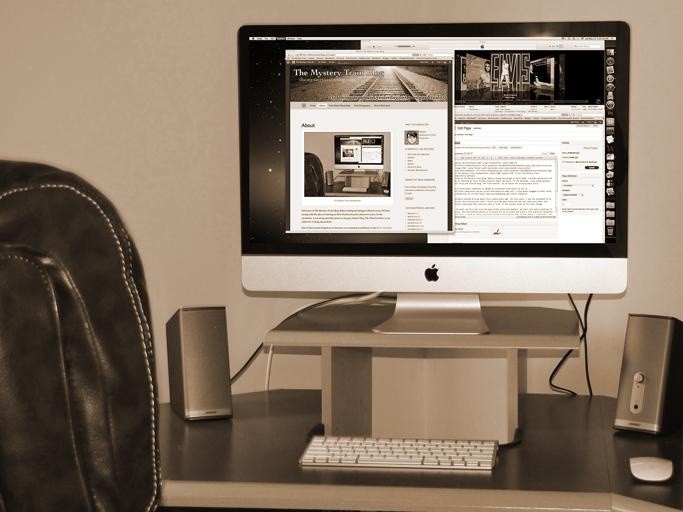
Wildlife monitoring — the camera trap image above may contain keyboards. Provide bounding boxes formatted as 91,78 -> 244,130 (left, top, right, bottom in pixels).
299,434 -> 500,470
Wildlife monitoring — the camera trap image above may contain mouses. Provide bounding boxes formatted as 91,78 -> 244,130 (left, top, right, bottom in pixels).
628,456 -> 674,482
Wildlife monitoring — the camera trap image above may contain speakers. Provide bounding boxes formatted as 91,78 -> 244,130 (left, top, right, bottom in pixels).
166,305 -> 233,420
613,311 -> 683,436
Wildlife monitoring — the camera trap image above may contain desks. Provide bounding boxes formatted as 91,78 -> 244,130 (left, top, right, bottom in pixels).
153,386 -> 683,511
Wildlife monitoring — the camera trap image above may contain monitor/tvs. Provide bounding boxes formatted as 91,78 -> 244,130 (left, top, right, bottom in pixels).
235,20 -> 630,337
